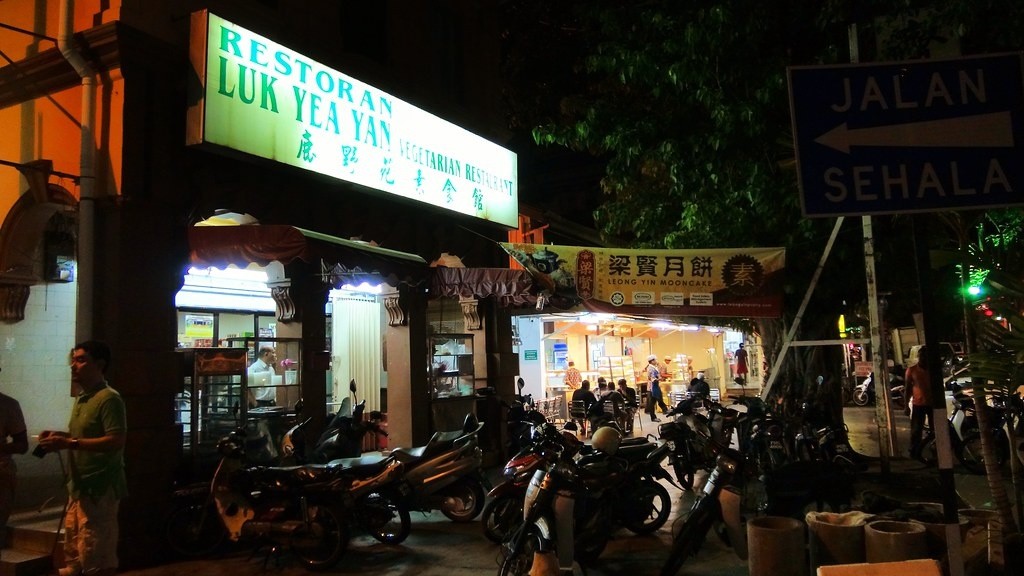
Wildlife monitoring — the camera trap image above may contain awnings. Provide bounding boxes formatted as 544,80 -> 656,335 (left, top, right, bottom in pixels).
429,267 -> 557,307
185,225 -> 428,289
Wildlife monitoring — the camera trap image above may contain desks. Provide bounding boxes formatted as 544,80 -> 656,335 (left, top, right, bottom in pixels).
637,382 -> 657,409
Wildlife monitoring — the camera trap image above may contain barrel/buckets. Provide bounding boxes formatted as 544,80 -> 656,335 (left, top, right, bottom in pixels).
865,520 -> 926,562
958,509 -> 1000,528
746,517 -> 808,576
806,513 -> 877,576
907,503 -> 970,550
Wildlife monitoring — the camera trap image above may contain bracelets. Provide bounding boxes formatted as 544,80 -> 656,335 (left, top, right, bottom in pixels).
70,438 -> 79,449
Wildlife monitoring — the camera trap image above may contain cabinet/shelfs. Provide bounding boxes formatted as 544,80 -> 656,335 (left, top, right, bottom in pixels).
427,333 -> 477,432
598,355 -> 637,395
225,336 -> 306,420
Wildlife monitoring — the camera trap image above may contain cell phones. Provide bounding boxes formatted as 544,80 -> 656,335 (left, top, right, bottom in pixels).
33,444 -> 47,459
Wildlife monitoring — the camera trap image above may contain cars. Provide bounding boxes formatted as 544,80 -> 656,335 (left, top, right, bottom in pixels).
903,340 -> 1005,430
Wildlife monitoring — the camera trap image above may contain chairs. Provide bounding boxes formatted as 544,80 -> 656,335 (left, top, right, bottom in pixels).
530,387 -> 720,441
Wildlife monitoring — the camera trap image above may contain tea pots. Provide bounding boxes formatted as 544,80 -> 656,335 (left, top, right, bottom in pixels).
526,247 -> 565,275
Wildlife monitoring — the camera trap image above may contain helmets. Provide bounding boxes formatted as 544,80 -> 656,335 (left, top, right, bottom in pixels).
229,458 -> 266,495
687,380 -> 710,395
592,426 -> 623,456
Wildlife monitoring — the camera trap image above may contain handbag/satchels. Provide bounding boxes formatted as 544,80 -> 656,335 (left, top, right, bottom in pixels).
564,422 -> 577,430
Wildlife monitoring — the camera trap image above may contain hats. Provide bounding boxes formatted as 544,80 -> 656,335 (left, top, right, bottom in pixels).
687,356 -> 693,361
665,356 -> 671,360
648,354 -> 657,361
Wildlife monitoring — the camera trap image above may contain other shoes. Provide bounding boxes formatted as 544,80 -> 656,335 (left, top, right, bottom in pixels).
581,432 -> 586,435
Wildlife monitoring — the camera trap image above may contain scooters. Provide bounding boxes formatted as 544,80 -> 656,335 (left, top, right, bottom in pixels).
171,376 -> 863,576
853,371 -> 906,408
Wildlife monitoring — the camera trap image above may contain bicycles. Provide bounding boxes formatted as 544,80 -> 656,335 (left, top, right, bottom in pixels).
918,374 -> 1007,474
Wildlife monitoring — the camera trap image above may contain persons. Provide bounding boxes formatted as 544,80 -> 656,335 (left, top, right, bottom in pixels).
0,368 -> 29,576
903,345 -> 933,463
733,343 -> 748,387
686,356 -> 693,381
570,376 -> 636,435
564,361 -> 582,389
686,373 -> 710,400
38,341 -> 128,576
642,354 -> 673,422
242,345 -> 277,408
